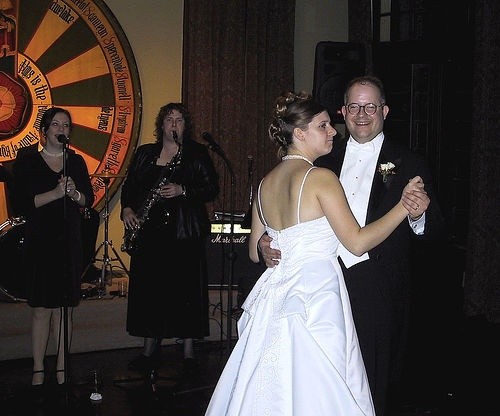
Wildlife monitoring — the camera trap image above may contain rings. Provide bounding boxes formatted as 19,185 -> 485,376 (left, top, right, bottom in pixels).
415,204 -> 420,210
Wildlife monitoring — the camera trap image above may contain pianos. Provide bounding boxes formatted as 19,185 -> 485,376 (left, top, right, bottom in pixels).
208,209 -> 253,247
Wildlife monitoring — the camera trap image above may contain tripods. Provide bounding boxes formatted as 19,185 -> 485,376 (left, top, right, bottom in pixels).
79,178 -> 130,298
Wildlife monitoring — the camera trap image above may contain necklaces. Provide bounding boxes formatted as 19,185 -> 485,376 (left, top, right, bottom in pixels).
42,149 -> 65,158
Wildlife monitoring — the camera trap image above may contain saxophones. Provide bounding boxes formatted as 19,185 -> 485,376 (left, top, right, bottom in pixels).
121,130 -> 181,253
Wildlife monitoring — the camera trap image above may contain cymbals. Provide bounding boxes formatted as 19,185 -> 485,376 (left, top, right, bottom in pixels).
88,174 -> 127,179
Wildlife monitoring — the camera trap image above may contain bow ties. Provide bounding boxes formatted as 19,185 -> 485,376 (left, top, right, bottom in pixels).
349,142 -> 375,154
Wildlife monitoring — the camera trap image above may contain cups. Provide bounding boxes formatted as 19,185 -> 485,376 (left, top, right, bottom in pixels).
118,281 -> 127,296
88,368 -> 104,396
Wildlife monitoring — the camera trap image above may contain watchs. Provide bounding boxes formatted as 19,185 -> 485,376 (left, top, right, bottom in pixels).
70,190 -> 81,202
178,184 -> 186,199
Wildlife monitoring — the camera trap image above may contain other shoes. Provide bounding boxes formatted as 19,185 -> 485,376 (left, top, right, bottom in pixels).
55,373 -> 65,396
181,357 -> 200,384
28,370 -> 45,403
112,365 -> 158,390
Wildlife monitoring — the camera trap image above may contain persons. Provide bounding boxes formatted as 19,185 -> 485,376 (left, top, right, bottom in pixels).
0,107 -> 96,397
113,102 -> 218,387
257,74 -> 432,416
204,91 -> 426,416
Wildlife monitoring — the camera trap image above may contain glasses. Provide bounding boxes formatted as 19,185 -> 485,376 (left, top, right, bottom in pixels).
345,102 -> 383,116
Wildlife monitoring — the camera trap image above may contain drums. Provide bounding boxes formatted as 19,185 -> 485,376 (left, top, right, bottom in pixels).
80,208 -> 91,220
0,218 -> 31,302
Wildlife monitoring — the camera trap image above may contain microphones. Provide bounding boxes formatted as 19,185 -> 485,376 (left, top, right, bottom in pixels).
202,132 -> 225,156
58,134 -> 70,144
